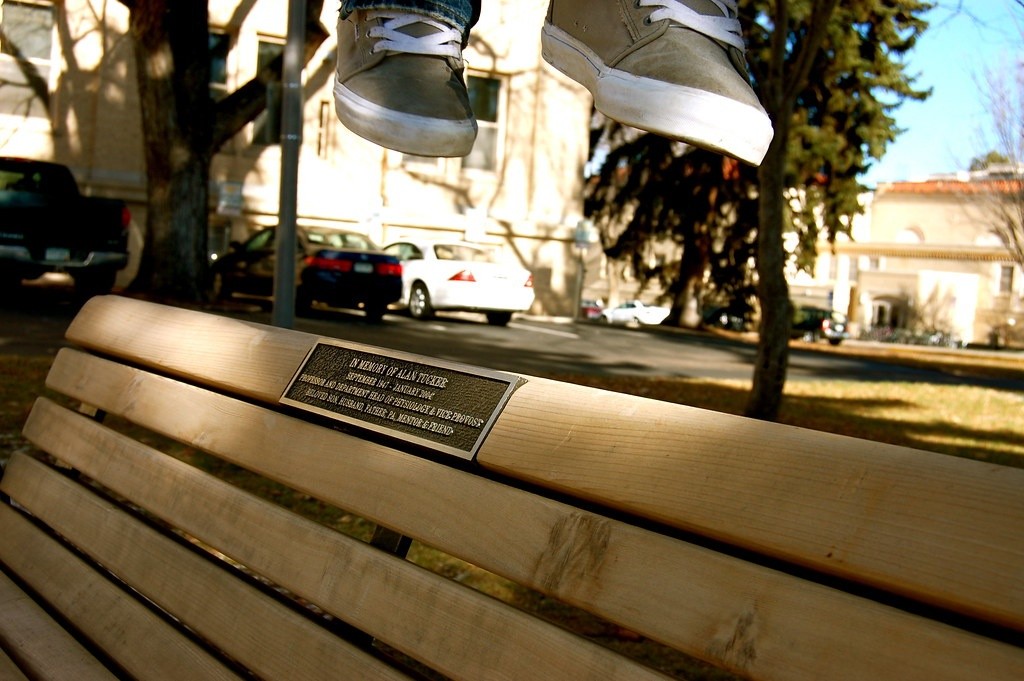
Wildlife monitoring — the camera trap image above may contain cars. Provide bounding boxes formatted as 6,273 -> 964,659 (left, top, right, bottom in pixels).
581,298 -> 750,331
210,224 -> 404,320
381,240 -> 536,327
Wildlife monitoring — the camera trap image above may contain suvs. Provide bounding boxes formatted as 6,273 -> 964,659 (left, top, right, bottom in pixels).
758,303 -> 850,346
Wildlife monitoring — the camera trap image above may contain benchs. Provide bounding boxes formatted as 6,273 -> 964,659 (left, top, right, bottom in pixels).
408,246 -> 424,260
0,296 -> 1024,681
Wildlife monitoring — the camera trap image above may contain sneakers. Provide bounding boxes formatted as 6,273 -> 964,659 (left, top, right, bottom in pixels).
539,0 -> 775,168
332,6 -> 479,157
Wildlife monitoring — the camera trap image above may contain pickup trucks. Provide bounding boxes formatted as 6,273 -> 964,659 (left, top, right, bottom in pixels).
0,156 -> 132,296
